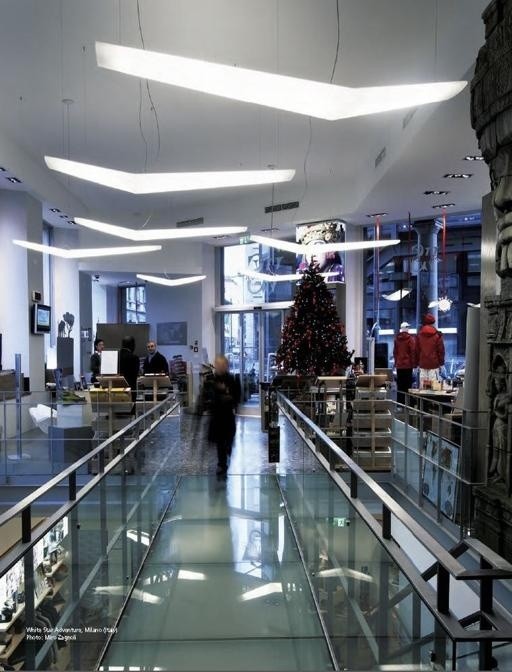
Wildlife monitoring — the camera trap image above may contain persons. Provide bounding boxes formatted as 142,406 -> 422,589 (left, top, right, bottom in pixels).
297,222 -> 345,284
90,339 -> 106,388
141,341 -> 170,402
392,321 -> 417,416
416,313 -> 445,389
193,354 -> 239,482
115,334 -> 141,418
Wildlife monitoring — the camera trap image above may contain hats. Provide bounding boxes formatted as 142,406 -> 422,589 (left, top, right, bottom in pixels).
400,321 -> 410,328
424,313 -> 435,324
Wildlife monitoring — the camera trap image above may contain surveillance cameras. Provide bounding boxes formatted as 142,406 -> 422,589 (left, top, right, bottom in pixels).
96,275 -> 99,278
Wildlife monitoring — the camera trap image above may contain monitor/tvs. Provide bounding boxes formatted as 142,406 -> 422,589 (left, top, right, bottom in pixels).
35,304 -> 52,334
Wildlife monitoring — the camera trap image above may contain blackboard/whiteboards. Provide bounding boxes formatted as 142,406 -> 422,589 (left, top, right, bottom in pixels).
97,323 -> 149,356
157,322 -> 187,345
